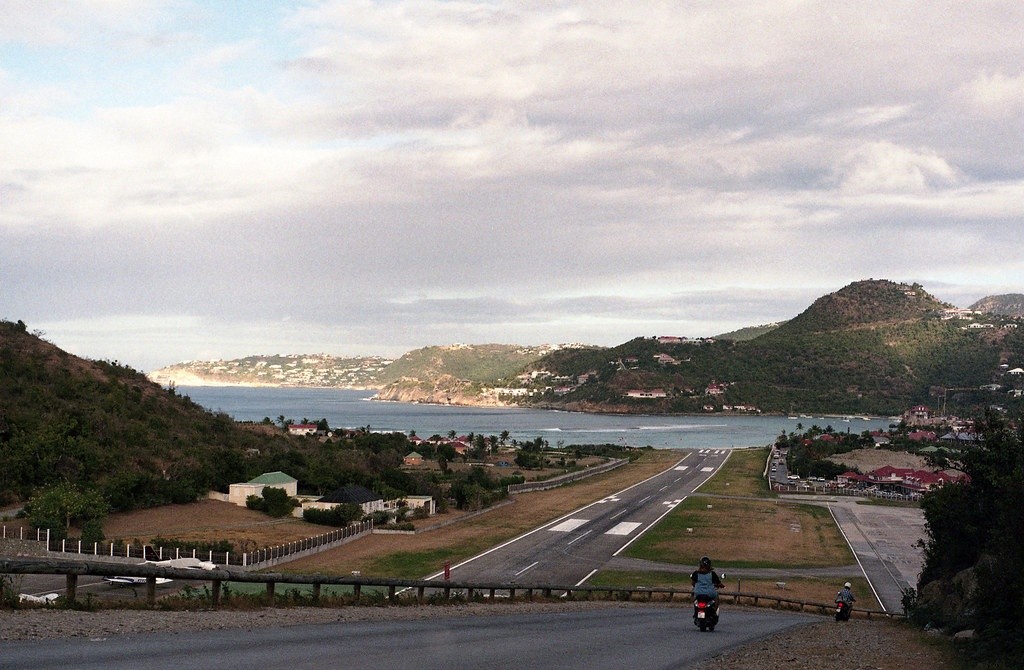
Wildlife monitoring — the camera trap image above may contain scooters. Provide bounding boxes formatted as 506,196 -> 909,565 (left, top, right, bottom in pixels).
834,591 -> 857,622
689,571 -> 726,633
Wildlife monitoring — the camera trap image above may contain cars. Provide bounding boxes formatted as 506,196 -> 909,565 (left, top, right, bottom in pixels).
772,450 -> 826,487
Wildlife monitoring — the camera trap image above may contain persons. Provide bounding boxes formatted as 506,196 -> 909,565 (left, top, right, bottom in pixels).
835,582 -> 856,618
691,557 -> 724,620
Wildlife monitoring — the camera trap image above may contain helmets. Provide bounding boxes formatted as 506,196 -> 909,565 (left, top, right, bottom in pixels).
845,582 -> 851,590
699,556 -> 711,568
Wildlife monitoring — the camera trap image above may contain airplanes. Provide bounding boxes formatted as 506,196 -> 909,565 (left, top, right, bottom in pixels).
102,558 -> 220,589
